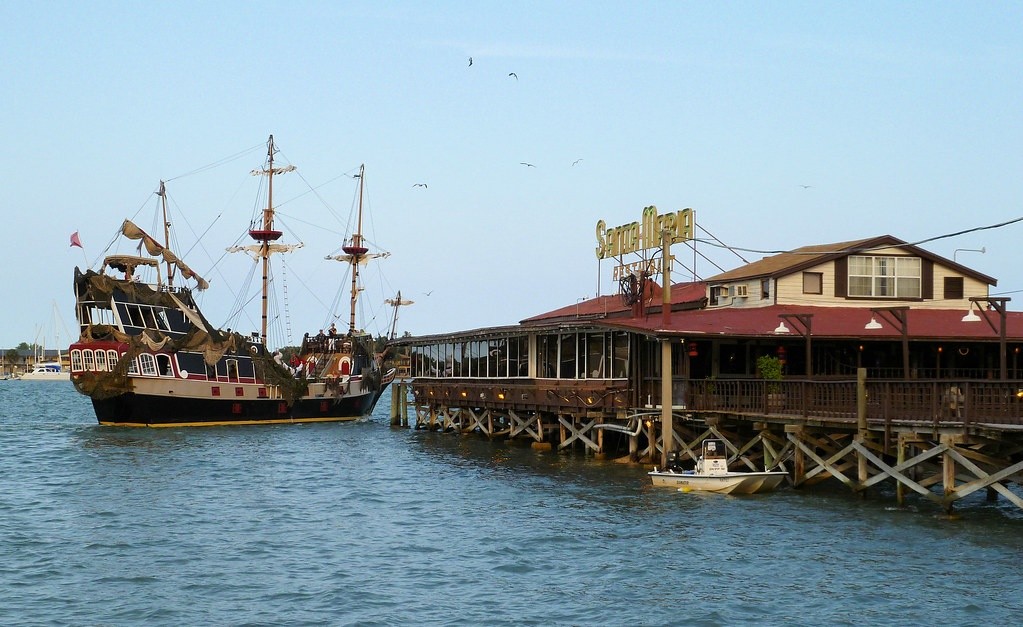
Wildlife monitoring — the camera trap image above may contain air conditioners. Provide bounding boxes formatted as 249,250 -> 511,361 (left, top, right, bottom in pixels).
728,285 -> 748,297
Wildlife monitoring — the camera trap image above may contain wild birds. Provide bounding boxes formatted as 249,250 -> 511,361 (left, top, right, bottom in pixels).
469,57 -> 473,66
413,184 -> 427,188
509,72 -> 518,79
797,185 -> 812,188
572,159 -> 583,166
520,163 -> 535,167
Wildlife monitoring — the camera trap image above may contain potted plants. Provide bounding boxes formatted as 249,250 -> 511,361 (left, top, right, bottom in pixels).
757,354 -> 787,413
695,376 -> 721,410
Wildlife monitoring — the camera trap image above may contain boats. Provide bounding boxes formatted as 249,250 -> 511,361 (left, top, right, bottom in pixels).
646,436 -> 791,495
20,344 -> 71,381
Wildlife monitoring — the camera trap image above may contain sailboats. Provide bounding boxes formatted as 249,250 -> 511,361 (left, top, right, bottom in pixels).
66,130 -> 414,427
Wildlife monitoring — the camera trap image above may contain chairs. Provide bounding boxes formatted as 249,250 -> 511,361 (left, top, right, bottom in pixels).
940,386 -> 964,417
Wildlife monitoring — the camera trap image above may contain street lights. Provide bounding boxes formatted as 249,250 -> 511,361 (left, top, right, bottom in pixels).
960,295 -> 1012,411
863,305 -> 913,418
772,312 -> 815,411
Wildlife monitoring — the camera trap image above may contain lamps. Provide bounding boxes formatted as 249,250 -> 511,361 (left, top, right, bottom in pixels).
415,390 -> 420,395
615,393 -> 624,403
430,391 -> 435,395
479,392 -> 485,398
499,390 -> 506,399
564,392 -> 572,402
588,394 -> 599,403
